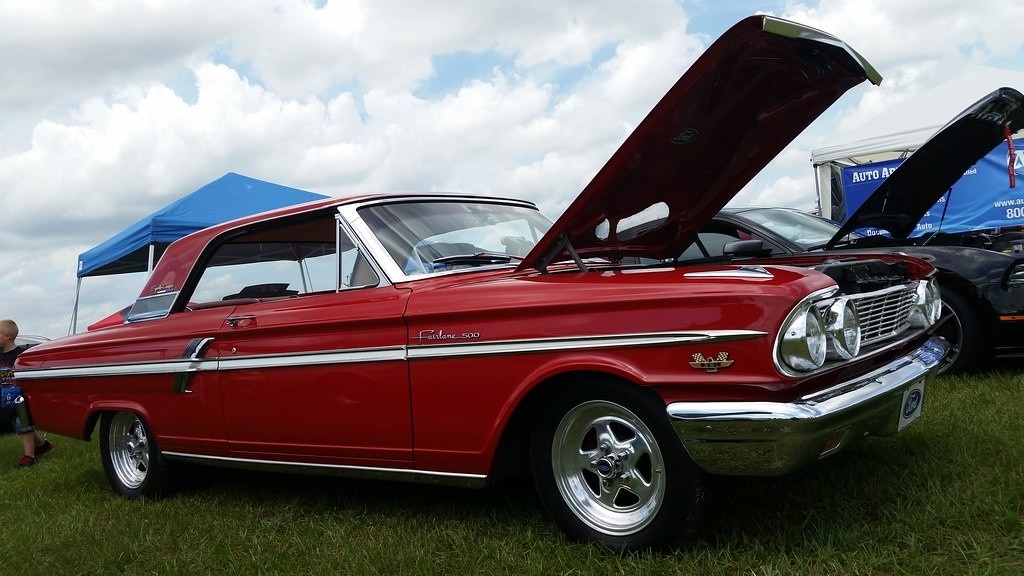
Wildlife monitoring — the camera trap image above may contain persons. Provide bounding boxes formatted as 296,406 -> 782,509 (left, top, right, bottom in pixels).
0,319 -> 54,469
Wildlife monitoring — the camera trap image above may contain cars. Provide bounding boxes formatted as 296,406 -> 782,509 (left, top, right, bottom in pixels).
605,85 -> 1024,375
11,14 -> 965,550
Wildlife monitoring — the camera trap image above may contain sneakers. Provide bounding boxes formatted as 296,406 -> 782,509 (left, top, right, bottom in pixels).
16,439 -> 55,467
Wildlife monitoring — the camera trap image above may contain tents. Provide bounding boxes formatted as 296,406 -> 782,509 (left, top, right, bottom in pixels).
69,172 -> 355,336
404,240 -> 453,274
